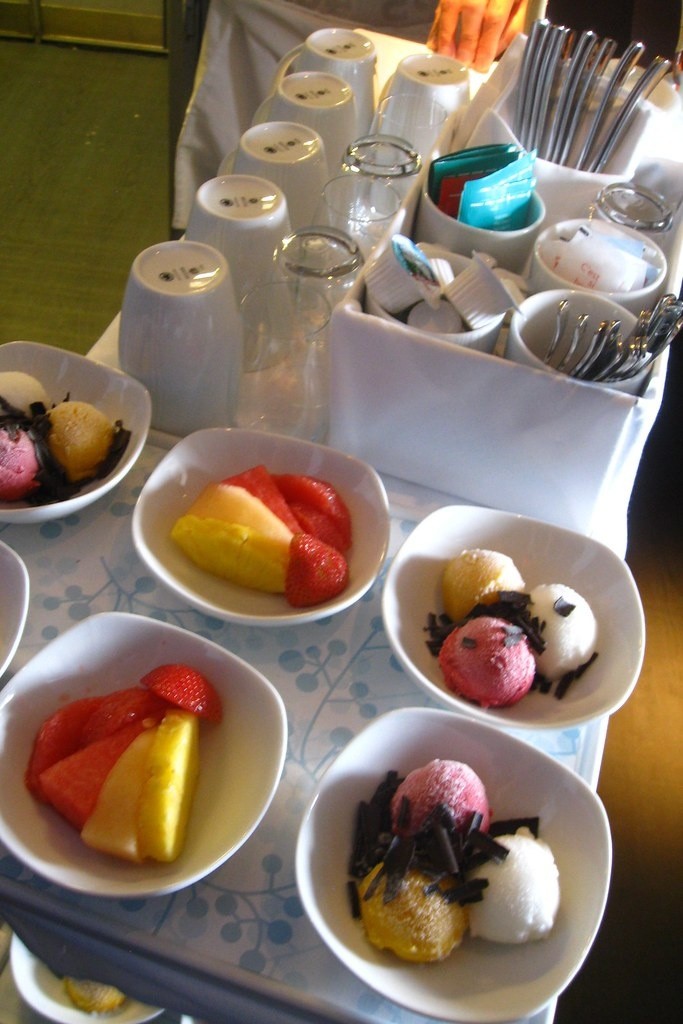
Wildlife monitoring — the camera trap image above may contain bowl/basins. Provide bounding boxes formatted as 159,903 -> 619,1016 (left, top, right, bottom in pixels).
331,171 -> 683,535
131,428 -> 389,627
0,612 -> 287,901
0,536 -> 30,679
380,505 -> 646,731
0,342 -> 152,525
295,706 -> 613,1024
467,53 -> 654,239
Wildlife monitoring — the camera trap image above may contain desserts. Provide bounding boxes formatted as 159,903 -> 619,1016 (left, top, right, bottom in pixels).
346,758 -> 559,965
424,550 -> 599,706
0,371 -> 131,506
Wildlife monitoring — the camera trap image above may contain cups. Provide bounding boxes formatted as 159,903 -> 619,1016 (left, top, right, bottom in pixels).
272,226 -> 365,310
363,247 -> 507,356
370,94 -> 448,166
232,282 -> 330,447
589,182 -> 674,251
527,217 -> 668,316
248,70 -> 360,175
414,175 -> 546,277
215,121 -> 326,225
379,52 -> 471,130
117,238 -> 240,436
502,289 -> 653,395
331,134 -> 421,200
270,27 -> 376,139
182,173 -> 291,301
312,174 -> 401,259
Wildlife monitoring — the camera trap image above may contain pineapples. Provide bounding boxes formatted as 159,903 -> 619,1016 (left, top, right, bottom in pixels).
173,484 -> 288,594
81,715 -> 200,859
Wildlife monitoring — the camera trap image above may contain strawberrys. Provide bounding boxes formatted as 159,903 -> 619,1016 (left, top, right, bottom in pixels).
224,465 -> 348,608
28,666 -> 224,830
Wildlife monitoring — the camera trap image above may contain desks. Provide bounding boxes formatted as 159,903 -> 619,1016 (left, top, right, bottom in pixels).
0,30 -> 634,1024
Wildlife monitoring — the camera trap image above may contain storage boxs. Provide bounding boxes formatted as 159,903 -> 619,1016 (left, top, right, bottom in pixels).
324,107 -> 683,534
